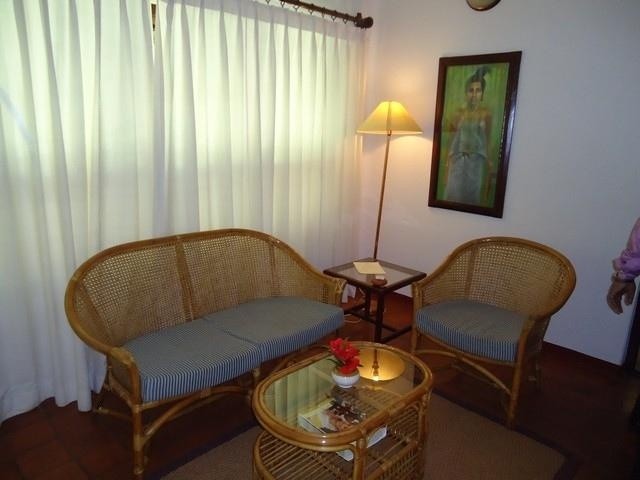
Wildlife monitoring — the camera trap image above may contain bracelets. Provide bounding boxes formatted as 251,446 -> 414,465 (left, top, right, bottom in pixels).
611,273 -> 634,283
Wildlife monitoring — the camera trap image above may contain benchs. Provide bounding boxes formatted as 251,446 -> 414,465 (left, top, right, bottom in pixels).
64,227 -> 347,480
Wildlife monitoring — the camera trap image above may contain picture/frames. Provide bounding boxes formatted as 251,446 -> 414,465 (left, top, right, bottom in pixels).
428,50 -> 522,219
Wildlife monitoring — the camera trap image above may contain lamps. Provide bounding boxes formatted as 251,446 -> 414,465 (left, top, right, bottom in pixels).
357,345 -> 407,381
355,100 -> 424,315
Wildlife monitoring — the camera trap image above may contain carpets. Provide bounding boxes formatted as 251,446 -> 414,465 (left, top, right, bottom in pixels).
154,377 -> 580,480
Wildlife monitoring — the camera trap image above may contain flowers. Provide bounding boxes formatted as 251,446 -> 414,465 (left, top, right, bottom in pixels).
322,338 -> 364,377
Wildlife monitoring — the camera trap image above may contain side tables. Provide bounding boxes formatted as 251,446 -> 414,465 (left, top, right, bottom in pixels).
322,256 -> 427,343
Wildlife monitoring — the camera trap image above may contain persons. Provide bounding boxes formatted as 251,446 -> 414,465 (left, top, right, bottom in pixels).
606,216 -> 640,314
443,73 -> 493,206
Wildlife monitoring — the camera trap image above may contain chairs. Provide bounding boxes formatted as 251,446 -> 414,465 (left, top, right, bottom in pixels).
409,236 -> 577,432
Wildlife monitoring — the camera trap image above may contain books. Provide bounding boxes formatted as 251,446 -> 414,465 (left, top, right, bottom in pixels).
298,402 -> 387,462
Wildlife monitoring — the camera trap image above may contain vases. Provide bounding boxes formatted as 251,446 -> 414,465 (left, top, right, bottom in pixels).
331,369 -> 360,388
